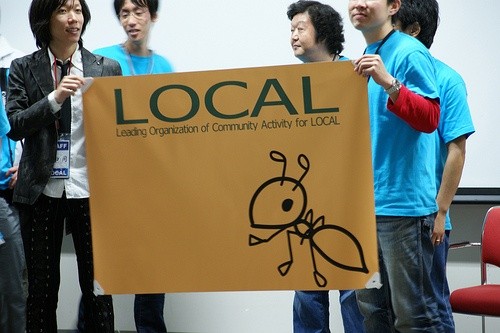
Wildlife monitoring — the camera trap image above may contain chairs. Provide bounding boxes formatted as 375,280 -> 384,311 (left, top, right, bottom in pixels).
449,205 -> 500,333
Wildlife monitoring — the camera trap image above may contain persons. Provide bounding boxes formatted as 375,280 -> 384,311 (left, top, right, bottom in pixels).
4,0 -> 124,333
90,0 -> 168,333
286,0 -> 366,333
388,1 -> 467,333
0,70 -> 28,333
346,1 -> 442,333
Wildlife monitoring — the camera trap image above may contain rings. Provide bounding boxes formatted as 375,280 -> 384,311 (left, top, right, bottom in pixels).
435,239 -> 442,242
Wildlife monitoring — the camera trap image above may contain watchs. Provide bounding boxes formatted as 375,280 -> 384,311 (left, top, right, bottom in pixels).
384,78 -> 402,95
